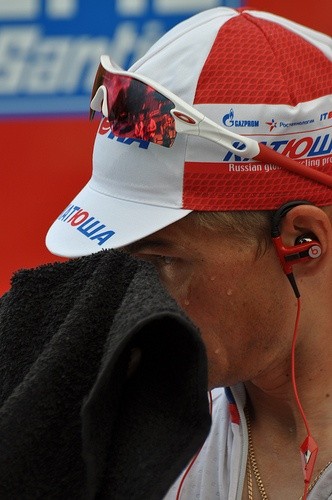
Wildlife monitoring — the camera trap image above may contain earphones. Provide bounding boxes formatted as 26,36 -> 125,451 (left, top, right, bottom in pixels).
270,201 -> 323,277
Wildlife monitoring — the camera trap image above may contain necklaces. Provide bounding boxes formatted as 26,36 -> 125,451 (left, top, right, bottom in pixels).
240,455 -> 256,499
246,409 -> 332,500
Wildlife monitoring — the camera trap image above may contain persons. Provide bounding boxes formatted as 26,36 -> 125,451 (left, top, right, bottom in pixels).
45,4 -> 331,500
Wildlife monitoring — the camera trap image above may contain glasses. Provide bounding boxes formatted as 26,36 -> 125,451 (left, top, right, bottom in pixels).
89,54 -> 332,189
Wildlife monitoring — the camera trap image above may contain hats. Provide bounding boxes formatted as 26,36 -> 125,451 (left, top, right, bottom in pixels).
45,6 -> 331,259
